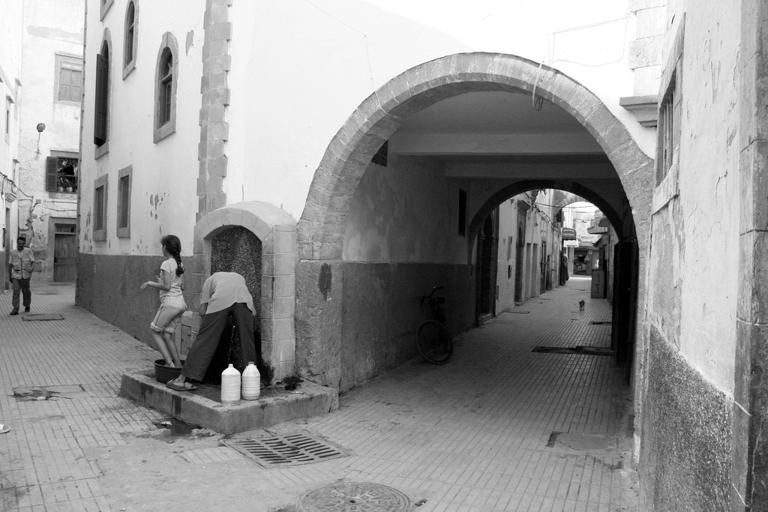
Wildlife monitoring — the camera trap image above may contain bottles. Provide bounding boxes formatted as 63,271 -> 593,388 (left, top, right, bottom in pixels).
221,362 -> 260,402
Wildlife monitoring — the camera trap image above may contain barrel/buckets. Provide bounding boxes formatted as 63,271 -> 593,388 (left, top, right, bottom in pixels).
242,361 -> 261,401
221,363 -> 241,402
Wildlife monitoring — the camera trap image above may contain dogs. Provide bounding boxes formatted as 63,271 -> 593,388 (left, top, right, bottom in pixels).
579,299 -> 585,311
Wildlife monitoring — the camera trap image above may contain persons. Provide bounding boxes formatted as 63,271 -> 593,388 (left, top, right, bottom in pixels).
166,271 -> 257,392
140,234 -> 187,368
8,237 -> 35,315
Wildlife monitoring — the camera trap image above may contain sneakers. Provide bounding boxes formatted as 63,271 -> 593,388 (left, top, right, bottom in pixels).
167,378 -> 197,391
25,307 -> 30,312
10,309 -> 19,315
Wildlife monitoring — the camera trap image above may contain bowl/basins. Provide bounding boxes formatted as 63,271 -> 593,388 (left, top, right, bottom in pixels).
154,359 -> 186,381
